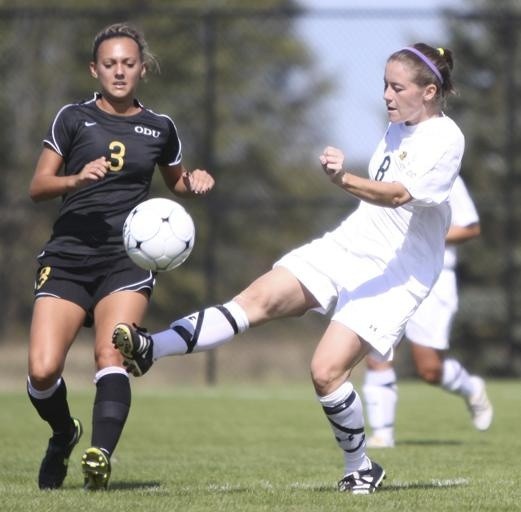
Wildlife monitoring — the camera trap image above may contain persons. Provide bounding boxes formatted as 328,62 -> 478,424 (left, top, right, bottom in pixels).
365,171 -> 496,450
111,42 -> 468,496
25,22 -> 217,493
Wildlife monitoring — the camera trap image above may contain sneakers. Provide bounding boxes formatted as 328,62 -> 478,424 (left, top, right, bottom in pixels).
39,418 -> 83,490
367,435 -> 394,448
465,377 -> 493,431
81,446 -> 111,490
112,322 -> 153,377
338,456 -> 386,495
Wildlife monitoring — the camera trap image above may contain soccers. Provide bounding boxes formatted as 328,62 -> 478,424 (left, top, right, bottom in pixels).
123,198 -> 195,272
